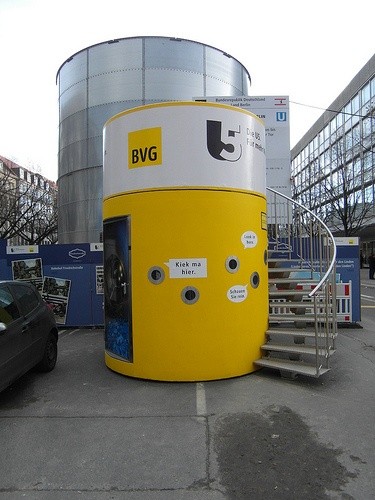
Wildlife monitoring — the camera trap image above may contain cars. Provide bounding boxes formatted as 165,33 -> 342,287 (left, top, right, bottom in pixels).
0,279 -> 58,393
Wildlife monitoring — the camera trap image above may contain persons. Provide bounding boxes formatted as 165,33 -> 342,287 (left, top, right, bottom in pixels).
368,253 -> 375,280
360,254 -> 364,269
